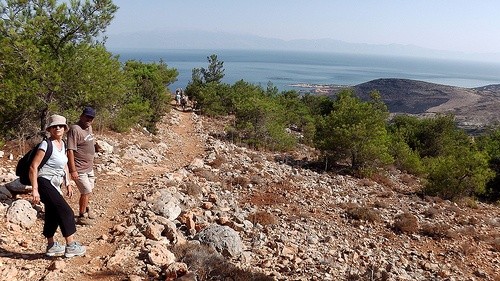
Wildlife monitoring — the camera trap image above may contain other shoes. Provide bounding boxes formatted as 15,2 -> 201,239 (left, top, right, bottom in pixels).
86,210 -> 97,219
77,212 -> 90,225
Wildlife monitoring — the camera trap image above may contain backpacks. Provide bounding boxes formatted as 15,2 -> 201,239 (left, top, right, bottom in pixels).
16,138 -> 66,186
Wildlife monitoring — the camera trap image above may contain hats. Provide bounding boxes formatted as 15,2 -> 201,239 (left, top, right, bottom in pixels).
83,107 -> 95,118
45,114 -> 69,133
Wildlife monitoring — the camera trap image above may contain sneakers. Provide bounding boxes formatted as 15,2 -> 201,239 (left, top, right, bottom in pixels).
64,241 -> 86,258
46,242 -> 65,257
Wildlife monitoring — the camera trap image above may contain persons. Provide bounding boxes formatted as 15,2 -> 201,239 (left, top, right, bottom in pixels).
66,107 -> 96,225
28,115 -> 86,257
175,87 -> 188,110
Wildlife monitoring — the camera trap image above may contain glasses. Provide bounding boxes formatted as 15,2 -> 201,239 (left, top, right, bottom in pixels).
52,125 -> 63,128
